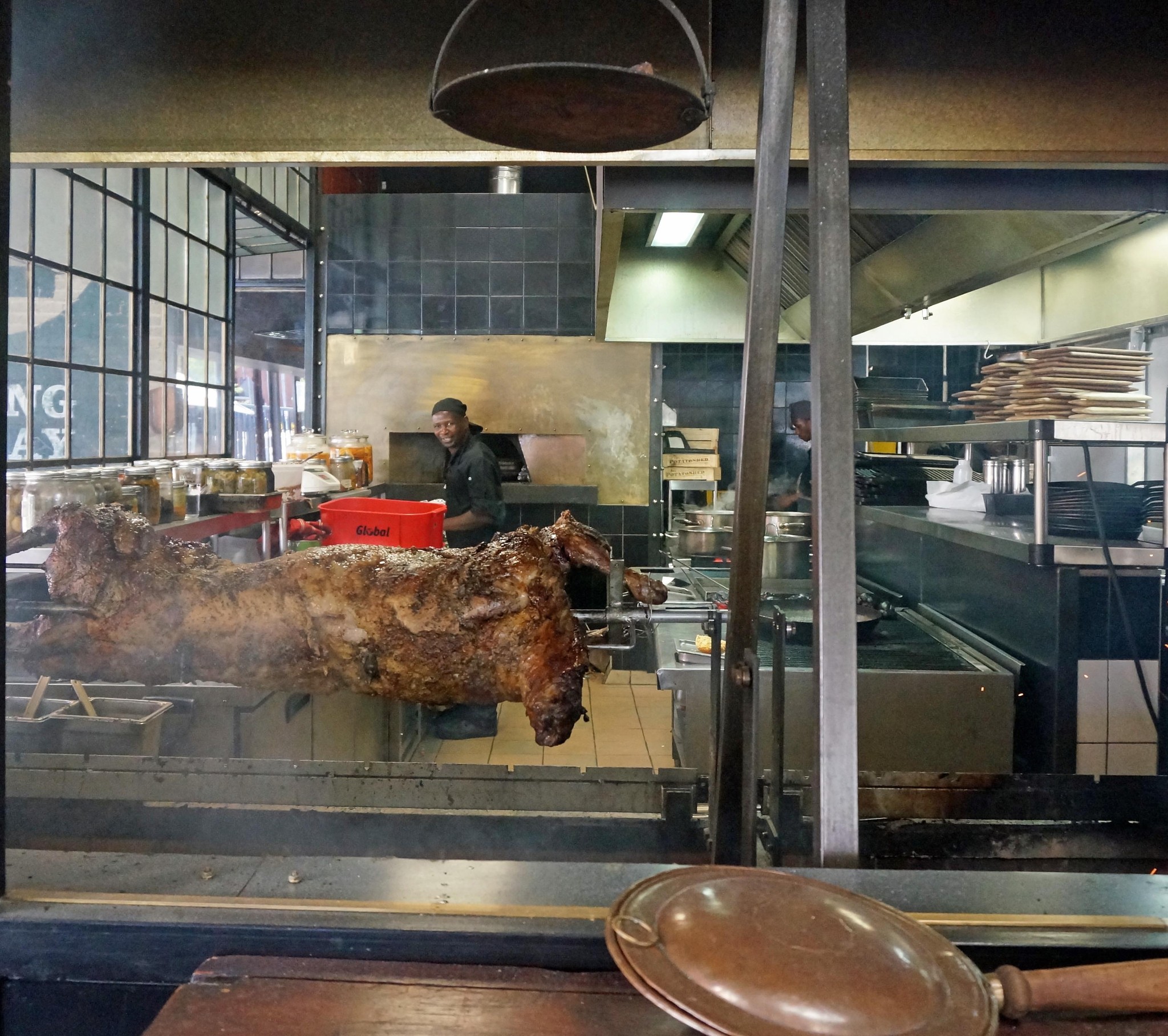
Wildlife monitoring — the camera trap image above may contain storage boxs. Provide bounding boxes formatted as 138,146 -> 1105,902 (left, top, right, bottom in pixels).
661,426 -> 722,481
317,496 -> 448,548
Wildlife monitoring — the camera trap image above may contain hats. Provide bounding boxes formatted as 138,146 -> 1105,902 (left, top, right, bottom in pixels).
431,398 -> 467,416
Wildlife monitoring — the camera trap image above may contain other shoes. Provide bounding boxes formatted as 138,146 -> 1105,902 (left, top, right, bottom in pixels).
432,707 -> 498,740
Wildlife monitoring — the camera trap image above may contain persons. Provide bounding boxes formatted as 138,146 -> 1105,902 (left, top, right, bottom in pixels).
773,400 -> 816,512
429,396 -> 505,740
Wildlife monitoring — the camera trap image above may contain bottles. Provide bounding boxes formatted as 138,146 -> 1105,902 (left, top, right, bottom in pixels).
982,459 -> 1030,495
953,459 -> 972,484
5,426 -> 374,540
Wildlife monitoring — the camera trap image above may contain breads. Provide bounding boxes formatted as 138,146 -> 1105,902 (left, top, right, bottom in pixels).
694,635 -> 726,655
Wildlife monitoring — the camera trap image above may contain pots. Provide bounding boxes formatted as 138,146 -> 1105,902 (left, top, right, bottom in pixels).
684,509 -> 734,527
758,593 -> 883,646
762,523 -> 811,579
664,525 -> 734,557
765,511 -> 812,538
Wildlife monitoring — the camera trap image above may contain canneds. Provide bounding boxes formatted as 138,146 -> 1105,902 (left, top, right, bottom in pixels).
7,457 -> 274,541
279,455 -> 356,493
